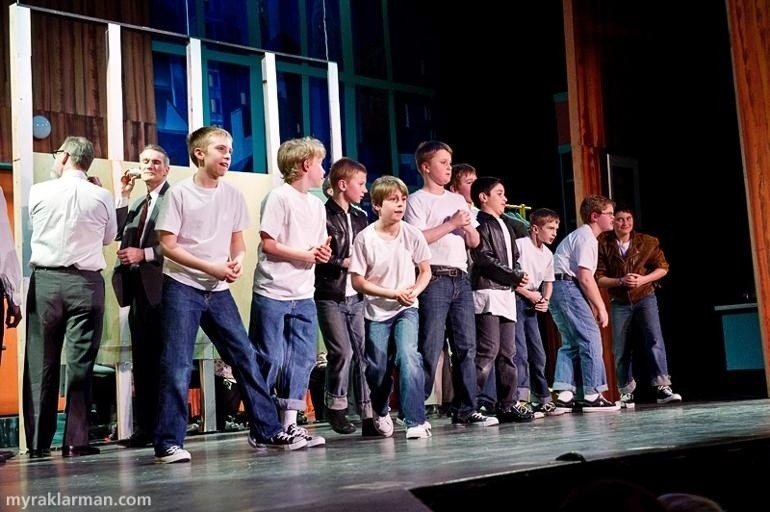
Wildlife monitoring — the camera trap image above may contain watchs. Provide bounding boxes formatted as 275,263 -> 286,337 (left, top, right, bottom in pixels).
618,277 -> 623,287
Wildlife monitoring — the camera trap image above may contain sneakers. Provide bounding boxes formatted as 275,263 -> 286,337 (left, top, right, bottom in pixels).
395,414 -> 432,431
655,384 -> 683,406
536,403 -> 565,417
153,444 -> 192,465
522,400 -> 544,419
452,410 -> 501,430
614,393 -> 636,411
554,396 -> 584,414
286,421 -> 326,449
372,405 -> 396,440
245,429 -> 309,452
404,426 -> 433,441
581,393 -> 620,413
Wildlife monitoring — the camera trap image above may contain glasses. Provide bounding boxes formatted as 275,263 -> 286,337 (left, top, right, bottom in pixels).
596,209 -> 614,218
53,149 -> 69,159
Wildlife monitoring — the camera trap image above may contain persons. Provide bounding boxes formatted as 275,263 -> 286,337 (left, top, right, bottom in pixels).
446,164 -> 482,228
24,136 -> 116,457
348,175 -> 431,441
250,139 -> 332,449
594,205 -> 681,408
404,142 -> 499,428
513,208 -> 561,421
0,187 -> 22,463
111,145 -> 175,447
154,127 -> 308,464
543,196 -> 624,414
313,159 -> 378,437
466,178 -> 534,423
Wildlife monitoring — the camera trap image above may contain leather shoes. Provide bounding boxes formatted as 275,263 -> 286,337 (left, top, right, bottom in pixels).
325,406 -> 358,435
495,405 -> 534,424
0,450 -> 15,459
60,442 -> 101,458
28,447 -> 53,460
115,427 -> 157,449
360,418 -> 382,438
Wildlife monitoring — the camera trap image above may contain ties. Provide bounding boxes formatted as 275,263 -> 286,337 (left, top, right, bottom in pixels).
134,190 -> 151,251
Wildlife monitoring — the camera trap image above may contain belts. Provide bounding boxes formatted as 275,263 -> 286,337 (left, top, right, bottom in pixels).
31,263 -> 82,272
553,272 -> 580,283
428,266 -> 472,280
